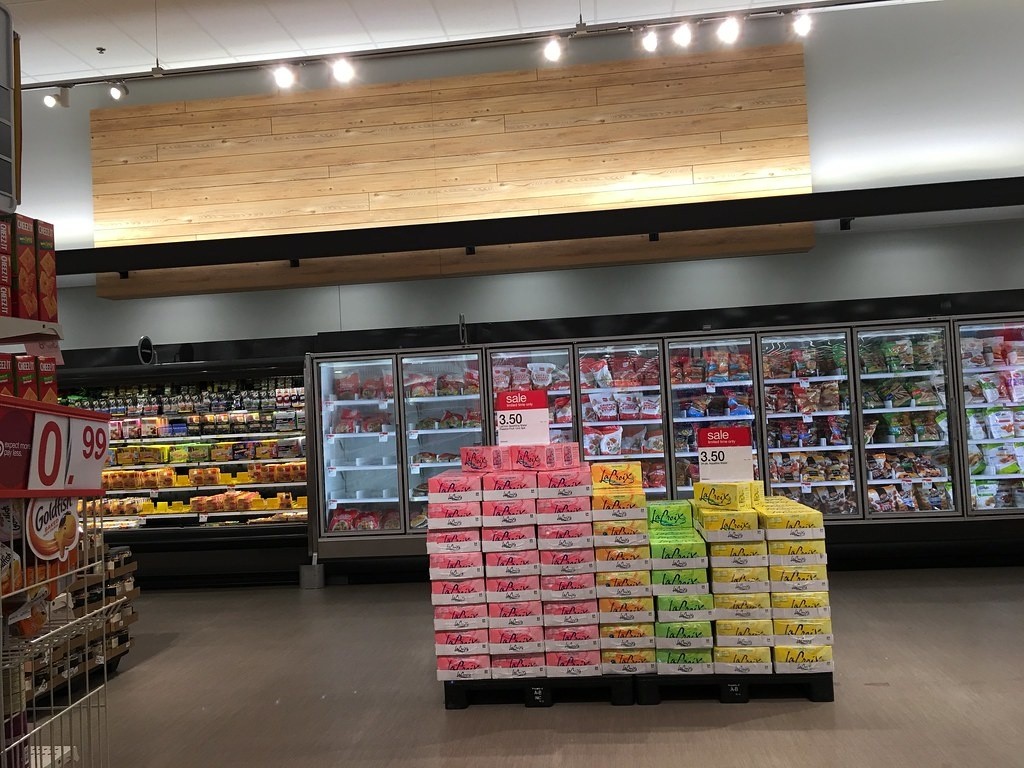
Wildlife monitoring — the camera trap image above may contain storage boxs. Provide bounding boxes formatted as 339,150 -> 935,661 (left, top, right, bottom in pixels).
21,549 -> 142,702
103,417 -> 307,467
0,209 -> 58,404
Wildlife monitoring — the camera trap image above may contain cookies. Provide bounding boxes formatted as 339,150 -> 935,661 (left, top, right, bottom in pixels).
42,387 -> 58,404
0,387 -> 12,395
23,386 -> 38,401
18,247 -> 57,320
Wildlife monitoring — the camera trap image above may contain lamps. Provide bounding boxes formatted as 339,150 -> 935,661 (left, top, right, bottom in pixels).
43,87 -> 70,108
107,79 -> 129,99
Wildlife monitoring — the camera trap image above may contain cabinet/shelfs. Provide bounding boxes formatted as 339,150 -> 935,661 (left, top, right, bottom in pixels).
0,316 -> 80,768
